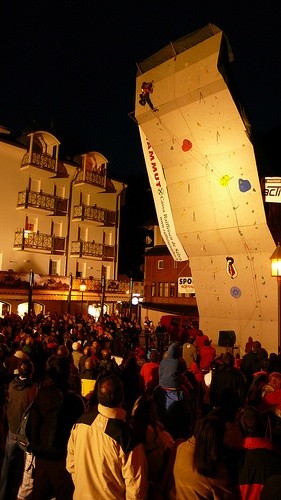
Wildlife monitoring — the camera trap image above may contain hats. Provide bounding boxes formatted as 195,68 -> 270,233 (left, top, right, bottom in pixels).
72,340 -> 81,350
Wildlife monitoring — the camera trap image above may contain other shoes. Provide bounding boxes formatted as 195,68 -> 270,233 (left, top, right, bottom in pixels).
153,109 -> 157,112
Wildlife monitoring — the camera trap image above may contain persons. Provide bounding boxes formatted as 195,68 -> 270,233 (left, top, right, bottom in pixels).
0,360 -> 86,500
172,414 -> 243,499
0,309 -> 281,438
134,399 -> 175,500
221,407 -> 281,500
155,428 -> 194,499
66,375 -> 148,500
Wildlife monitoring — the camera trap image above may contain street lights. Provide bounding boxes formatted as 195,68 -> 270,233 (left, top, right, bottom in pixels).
80,280 -> 87,314
270,242 -> 281,357
